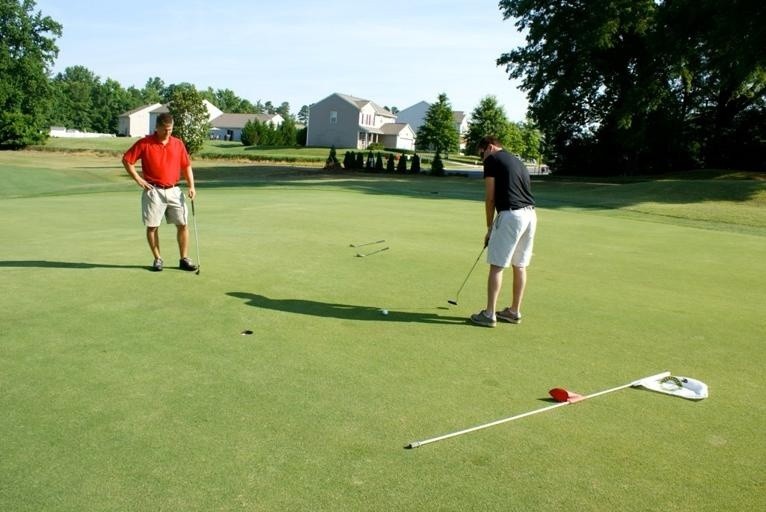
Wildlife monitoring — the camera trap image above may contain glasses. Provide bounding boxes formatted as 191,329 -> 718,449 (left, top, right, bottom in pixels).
480,146 -> 488,158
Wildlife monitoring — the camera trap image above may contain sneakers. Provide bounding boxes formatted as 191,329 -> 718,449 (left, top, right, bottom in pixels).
471,311 -> 497,327
180,258 -> 198,271
153,257 -> 163,271
496,308 -> 522,324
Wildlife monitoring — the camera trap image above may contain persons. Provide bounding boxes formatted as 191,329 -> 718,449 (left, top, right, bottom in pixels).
121,113 -> 198,272
470,136 -> 538,328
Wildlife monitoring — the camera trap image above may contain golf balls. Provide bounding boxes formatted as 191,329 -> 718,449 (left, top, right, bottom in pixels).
382,309 -> 388,315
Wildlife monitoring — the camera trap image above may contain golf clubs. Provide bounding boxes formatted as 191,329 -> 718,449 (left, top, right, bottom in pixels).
350,239 -> 385,247
189,195 -> 200,275
356,247 -> 389,257
448,238 -> 489,305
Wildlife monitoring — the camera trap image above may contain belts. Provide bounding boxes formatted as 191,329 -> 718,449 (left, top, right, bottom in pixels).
147,182 -> 182,190
